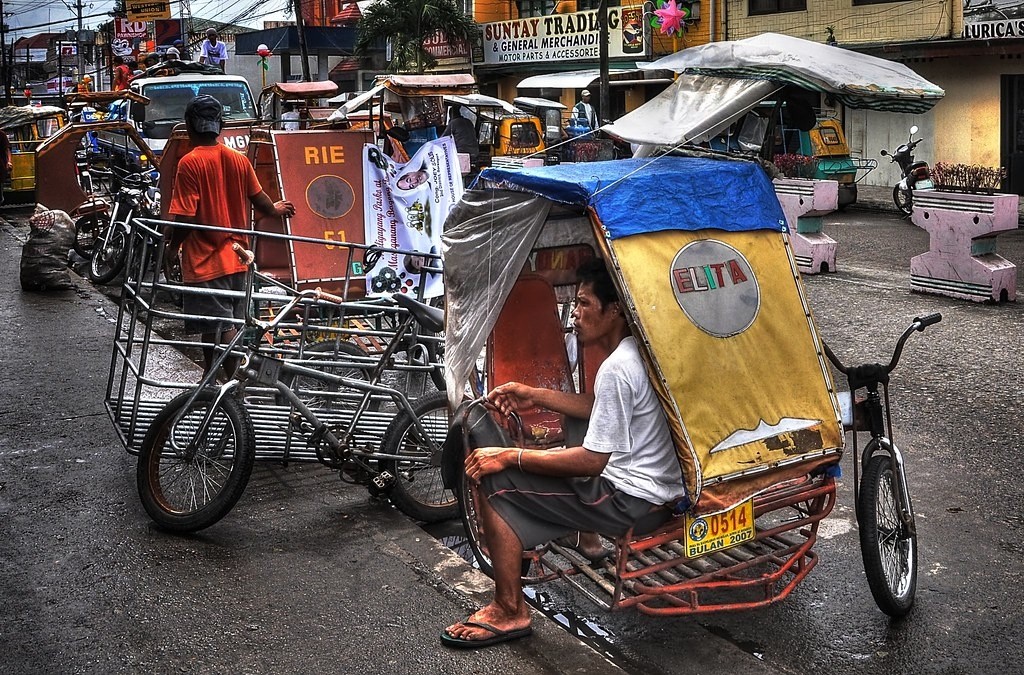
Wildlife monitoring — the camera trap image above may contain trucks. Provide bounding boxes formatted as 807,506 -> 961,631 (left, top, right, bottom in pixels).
129,61 -> 260,156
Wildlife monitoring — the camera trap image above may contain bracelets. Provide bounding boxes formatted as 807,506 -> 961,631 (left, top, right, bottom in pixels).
518,449 -> 525,473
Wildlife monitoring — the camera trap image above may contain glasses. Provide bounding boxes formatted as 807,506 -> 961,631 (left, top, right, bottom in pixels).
176,45 -> 184,49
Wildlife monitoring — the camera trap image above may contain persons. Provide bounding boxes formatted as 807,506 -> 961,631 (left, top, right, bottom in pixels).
111,40 -> 191,93
571,90 -> 600,131
440,257 -> 684,647
0,130 -> 13,203
199,28 -> 228,74
281,102 -> 300,130
154,96 -> 296,385
439,107 -> 479,192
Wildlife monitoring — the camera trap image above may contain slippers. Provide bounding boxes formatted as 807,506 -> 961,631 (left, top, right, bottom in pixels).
553,531 -> 611,560
439,615 -> 533,648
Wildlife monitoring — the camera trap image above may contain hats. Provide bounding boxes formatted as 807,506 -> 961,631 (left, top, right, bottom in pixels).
206,28 -> 219,39
166,46 -> 181,60
147,52 -> 159,63
580,89 -> 591,98
173,40 -> 185,47
185,95 -> 223,135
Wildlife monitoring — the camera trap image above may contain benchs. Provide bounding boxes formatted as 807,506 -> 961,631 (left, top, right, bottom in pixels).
255,216 -> 291,283
74,196 -> 110,215
484,275 -> 609,449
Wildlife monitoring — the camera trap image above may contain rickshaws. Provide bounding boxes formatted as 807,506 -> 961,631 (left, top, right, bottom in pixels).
440,155 -> 942,617
0,69 -> 567,262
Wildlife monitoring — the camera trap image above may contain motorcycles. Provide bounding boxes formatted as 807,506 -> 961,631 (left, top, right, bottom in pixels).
71,159 -> 184,302
705,100 -> 878,209
880,125 -> 936,219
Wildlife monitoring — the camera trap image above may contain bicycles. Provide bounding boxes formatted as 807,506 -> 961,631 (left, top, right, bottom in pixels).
134,244 -> 473,533
